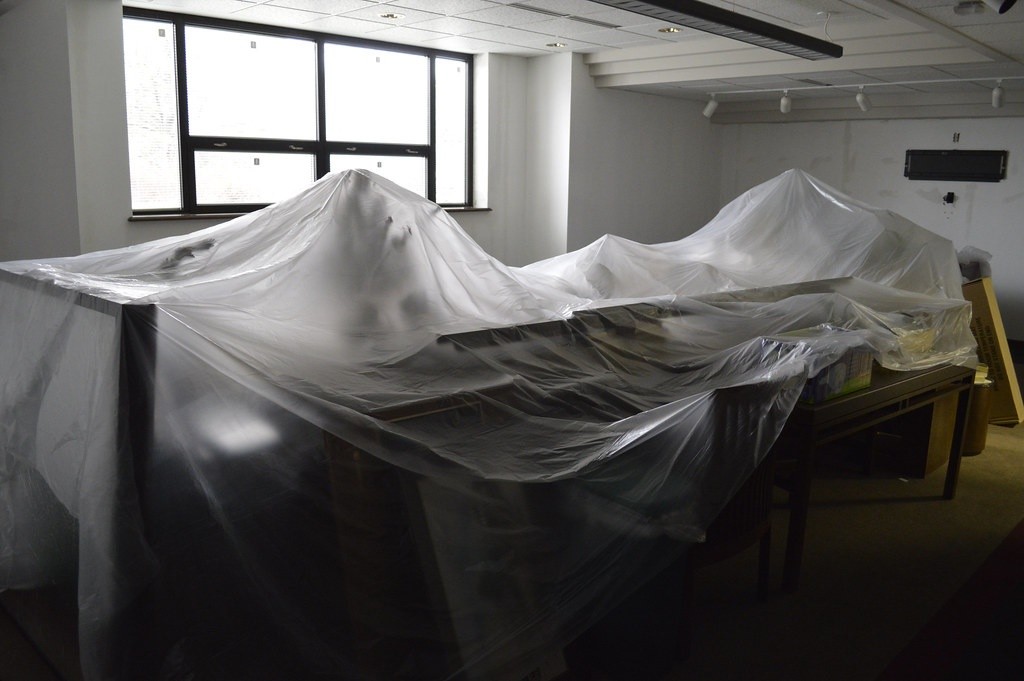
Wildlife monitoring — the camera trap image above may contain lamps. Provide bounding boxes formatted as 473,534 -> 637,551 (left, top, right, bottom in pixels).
981,0 -> 1019,13
855,85 -> 872,111
991,78 -> 1006,107
702,93 -> 719,118
780,90 -> 792,113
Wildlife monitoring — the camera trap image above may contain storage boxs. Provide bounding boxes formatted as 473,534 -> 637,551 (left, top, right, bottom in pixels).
961,277 -> 1024,425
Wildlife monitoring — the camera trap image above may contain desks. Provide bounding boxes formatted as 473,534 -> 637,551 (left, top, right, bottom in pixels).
783,362 -> 975,592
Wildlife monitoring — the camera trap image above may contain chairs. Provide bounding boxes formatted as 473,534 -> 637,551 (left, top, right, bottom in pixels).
666,378 -> 782,660
